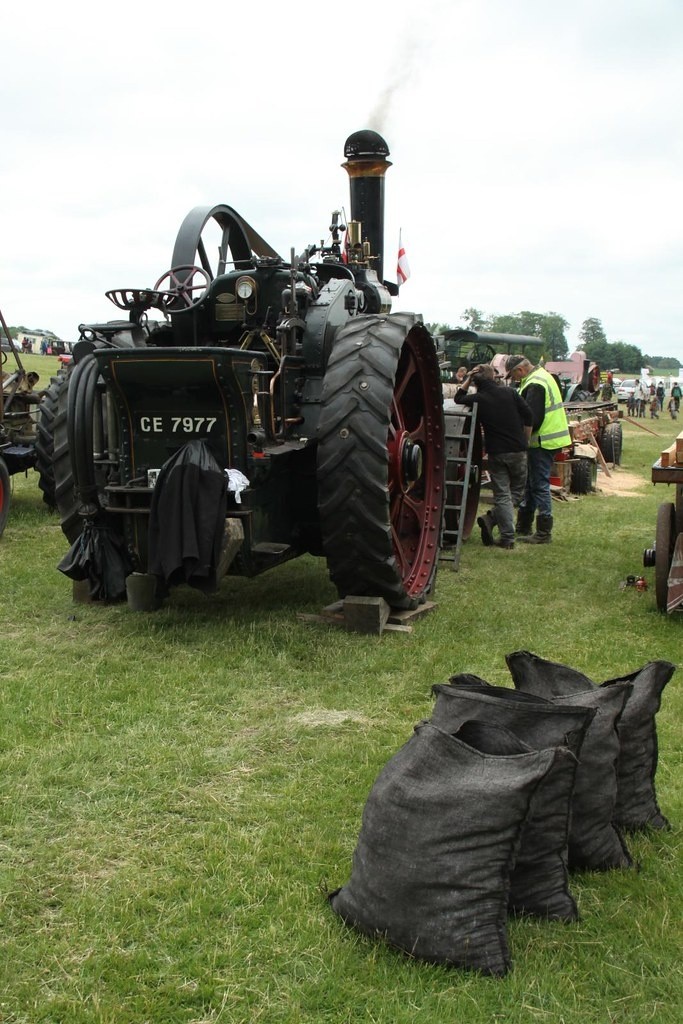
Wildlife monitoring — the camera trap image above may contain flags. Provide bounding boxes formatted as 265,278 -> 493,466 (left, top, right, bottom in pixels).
397,235 -> 411,286
342,227 -> 350,264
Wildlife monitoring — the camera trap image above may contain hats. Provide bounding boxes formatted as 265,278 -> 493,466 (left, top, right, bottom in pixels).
503,354 -> 525,379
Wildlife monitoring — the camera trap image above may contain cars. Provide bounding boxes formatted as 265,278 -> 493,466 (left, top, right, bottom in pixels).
0,337 -> 21,352
616,379 -> 651,403
598,378 -> 623,392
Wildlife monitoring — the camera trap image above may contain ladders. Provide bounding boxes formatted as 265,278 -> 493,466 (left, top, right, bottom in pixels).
439,402 -> 478,570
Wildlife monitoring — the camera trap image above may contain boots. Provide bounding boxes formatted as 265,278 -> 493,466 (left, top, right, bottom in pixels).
517,515 -> 552,544
515,509 -> 534,536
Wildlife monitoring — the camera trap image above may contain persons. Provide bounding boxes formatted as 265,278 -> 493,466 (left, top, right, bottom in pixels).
505,355 -> 572,544
601,369 -> 682,421
453,365 -> 534,550
41,341 -> 46,355
21,336 -> 32,354
350,243 -> 368,264
449,367 -> 467,384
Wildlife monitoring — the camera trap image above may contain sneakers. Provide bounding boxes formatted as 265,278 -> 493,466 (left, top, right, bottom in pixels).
477,515 -> 496,546
493,537 -> 513,547
